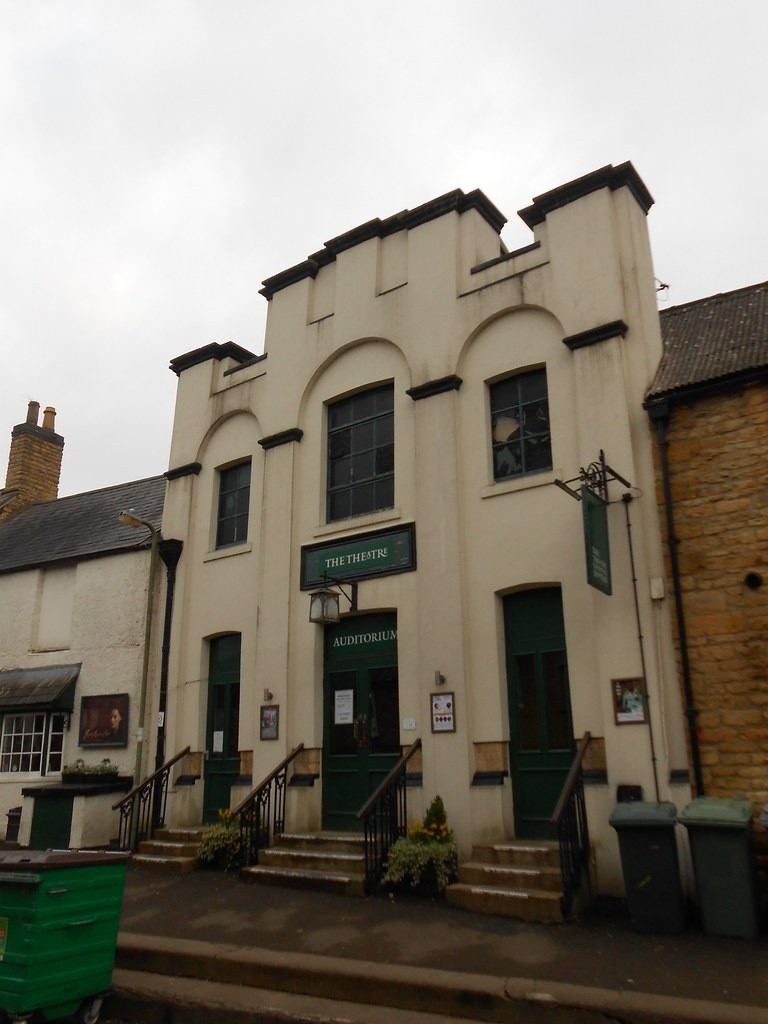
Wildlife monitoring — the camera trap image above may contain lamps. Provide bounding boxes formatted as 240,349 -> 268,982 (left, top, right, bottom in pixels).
306,572 -> 358,624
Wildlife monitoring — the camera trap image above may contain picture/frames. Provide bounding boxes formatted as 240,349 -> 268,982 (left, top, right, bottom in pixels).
610,679 -> 649,724
429,692 -> 457,733
259,704 -> 279,740
78,693 -> 127,748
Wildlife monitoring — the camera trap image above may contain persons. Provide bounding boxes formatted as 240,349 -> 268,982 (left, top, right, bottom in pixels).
104,700 -> 123,743
622,682 -> 642,713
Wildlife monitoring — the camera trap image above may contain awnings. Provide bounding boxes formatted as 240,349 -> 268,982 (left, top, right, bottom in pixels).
0,663 -> 81,707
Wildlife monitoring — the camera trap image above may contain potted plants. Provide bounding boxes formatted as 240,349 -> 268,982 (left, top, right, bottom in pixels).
62,765 -> 120,784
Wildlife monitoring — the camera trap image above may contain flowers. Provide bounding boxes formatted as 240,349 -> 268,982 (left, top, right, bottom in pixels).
382,822 -> 460,892
200,810 -> 258,865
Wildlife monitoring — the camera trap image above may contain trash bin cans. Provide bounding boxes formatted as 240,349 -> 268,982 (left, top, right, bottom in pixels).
679,797 -> 758,938
607,800 -> 681,932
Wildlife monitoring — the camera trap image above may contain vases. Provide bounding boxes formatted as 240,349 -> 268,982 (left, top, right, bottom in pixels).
403,865 -> 436,899
213,849 -> 245,873
